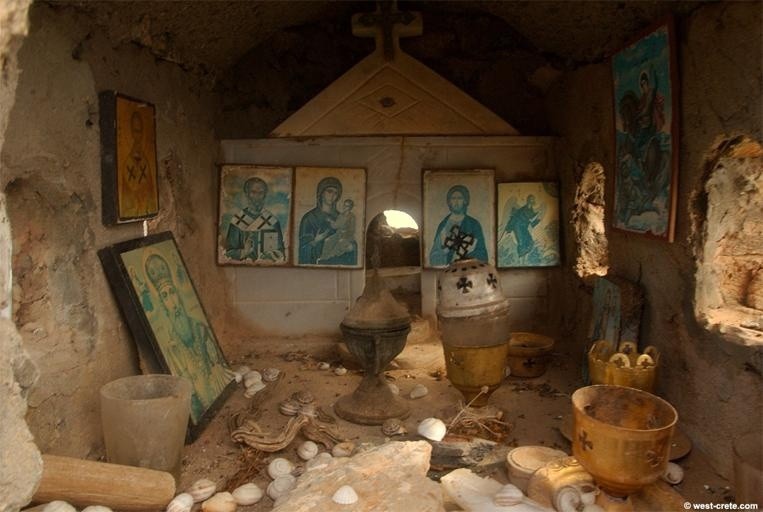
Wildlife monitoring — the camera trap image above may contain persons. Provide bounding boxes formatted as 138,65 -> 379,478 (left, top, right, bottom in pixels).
505,194 -> 546,267
635,68 -> 666,132
119,110 -> 158,218
320,199 -> 357,260
224,176 -> 285,263
297,176 -> 358,266
426,184 -> 489,267
171,254 -> 187,288
144,254 -> 236,427
128,265 -> 153,314
614,90 -> 673,238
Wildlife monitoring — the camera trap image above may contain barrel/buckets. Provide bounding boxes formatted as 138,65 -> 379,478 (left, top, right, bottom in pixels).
730,426 -> 763,512
508,333 -> 555,377
102,374 -> 191,491
587,340 -> 664,394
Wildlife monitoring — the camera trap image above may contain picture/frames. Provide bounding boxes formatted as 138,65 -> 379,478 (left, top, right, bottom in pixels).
494,180 -> 564,269
217,162 -> 294,268
97,88 -> 160,225
292,165 -> 369,270
96,230 -> 241,440
420,167 -> 497,270
607,16 -> 681,244
